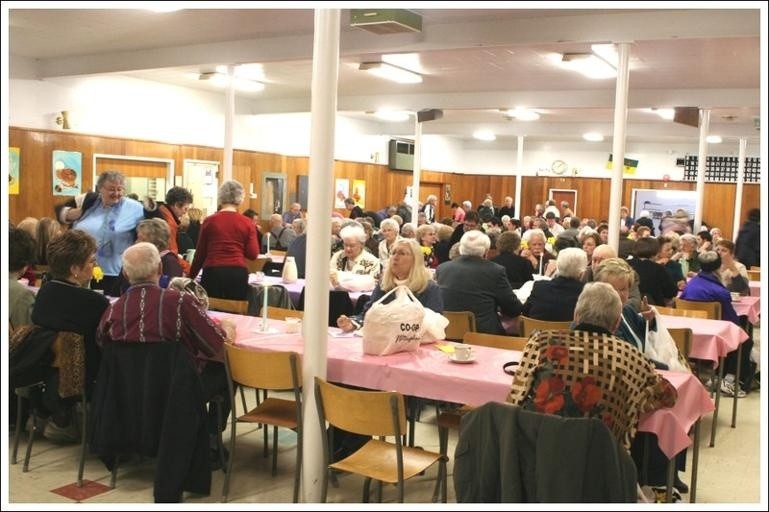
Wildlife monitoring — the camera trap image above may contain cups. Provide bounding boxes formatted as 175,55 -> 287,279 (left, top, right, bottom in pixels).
454,345 -> 471,359
285,317 -> 301,333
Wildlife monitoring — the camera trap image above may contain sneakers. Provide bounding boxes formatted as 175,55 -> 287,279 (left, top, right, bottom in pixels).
720,378 -> 746,398
44,416 -> 81,441
25,414 -> 47,432
705,375 -> 719,392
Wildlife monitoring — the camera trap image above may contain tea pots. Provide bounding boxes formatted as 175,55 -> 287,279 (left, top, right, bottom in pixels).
183,249 -> 196,265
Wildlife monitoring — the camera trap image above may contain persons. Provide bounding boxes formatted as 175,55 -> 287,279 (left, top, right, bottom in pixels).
331,193 -> 761,342
9,226 -> 82,444
17,172 -> 306,300
680,251 -> 750,399
503,278 -> 681,456
96,239 -> 238,473
568,258 -> 693,494
324,238 -> 446,474
32,232 -> 108,348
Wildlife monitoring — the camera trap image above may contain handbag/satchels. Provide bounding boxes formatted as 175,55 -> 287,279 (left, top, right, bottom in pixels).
644,304 -> 692,370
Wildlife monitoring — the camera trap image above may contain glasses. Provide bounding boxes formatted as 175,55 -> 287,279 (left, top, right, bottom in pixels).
101,183 -> 125,192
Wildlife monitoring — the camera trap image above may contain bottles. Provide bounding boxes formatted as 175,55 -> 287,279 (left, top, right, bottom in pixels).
282,256 -> 298,284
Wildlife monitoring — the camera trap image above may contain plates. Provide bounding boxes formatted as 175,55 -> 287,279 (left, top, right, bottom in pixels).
449,356 -> 476,362
